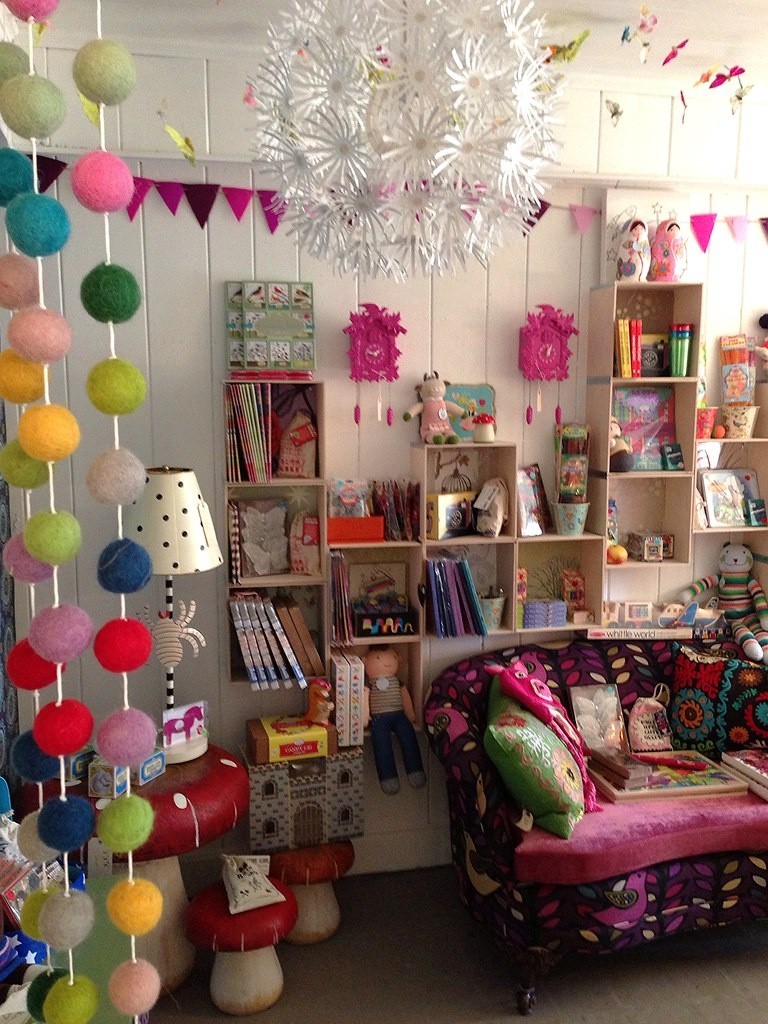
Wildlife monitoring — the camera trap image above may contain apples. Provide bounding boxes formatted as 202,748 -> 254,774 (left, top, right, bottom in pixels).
607,544 -> 628,564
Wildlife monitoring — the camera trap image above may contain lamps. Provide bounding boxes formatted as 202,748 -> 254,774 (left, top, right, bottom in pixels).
246,0 -> 554,282
122,466 -> 223,766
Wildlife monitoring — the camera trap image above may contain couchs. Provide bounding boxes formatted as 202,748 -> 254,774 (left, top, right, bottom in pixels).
421,636 -> 768,1015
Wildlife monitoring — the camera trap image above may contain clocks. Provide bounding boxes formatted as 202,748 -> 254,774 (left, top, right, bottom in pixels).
518,304 -> 580,383
342,304 -> 407,384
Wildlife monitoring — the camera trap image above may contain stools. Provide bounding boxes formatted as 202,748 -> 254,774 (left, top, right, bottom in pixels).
180,877 -> 300,1015
267,838 -> 355,946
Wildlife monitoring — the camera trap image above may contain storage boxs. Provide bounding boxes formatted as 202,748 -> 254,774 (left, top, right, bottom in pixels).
574,626 -> 693,640
229,595 -> 366,854
600,185 -> 708,283
52,743 -> 166,798
426,490 -> 477,540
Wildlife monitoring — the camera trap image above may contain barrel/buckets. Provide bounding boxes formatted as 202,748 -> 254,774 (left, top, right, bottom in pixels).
698,404 -> 760,438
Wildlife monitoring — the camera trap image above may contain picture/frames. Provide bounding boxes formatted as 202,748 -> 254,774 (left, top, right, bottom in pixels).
583,750 -> 749,805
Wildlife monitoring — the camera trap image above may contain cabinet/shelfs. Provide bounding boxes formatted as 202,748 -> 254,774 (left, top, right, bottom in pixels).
227,282 -> 768,738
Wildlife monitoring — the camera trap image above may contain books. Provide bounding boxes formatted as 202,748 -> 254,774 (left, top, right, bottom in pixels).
425,548 -> 489,639
614,318 -> 694,377
228,589 -> 325,692
227,497 -> 320,587
225,382 -> 272,485
330,648 -> 365,747
330,550 -> 419,648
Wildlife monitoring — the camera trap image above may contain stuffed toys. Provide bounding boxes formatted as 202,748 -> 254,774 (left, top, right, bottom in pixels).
403,371 -> 469,445
610,415 -> 635,473
361,644 -> 426,795
678,541 -> 768,666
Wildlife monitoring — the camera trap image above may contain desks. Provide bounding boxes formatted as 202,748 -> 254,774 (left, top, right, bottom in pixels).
15,745 -> 251,997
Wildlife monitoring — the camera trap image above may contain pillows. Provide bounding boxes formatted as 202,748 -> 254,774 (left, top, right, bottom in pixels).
482,675 -> 585,840
667,642 -> 768,761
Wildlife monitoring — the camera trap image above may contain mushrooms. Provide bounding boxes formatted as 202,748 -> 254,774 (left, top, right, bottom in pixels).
15,742 -> 356,1015
